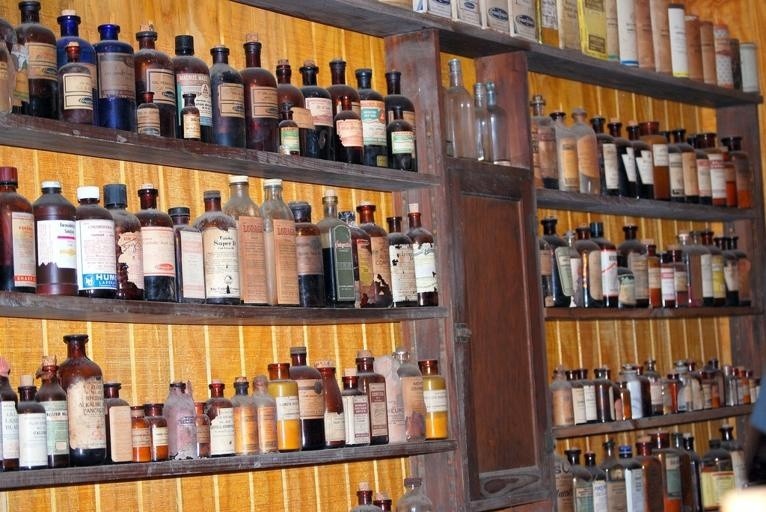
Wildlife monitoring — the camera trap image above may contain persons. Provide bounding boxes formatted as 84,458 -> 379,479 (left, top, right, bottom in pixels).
744,371 -> 765,483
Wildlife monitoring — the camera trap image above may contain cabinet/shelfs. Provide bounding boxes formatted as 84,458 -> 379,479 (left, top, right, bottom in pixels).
0,0 -> 766,512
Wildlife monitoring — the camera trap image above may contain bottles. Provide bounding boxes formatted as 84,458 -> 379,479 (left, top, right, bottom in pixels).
1,1 -> 761,213
1,156 -> 762,311
351,422 -> 762,509
393,0 -> 759,92
0,330 -> 740,465
